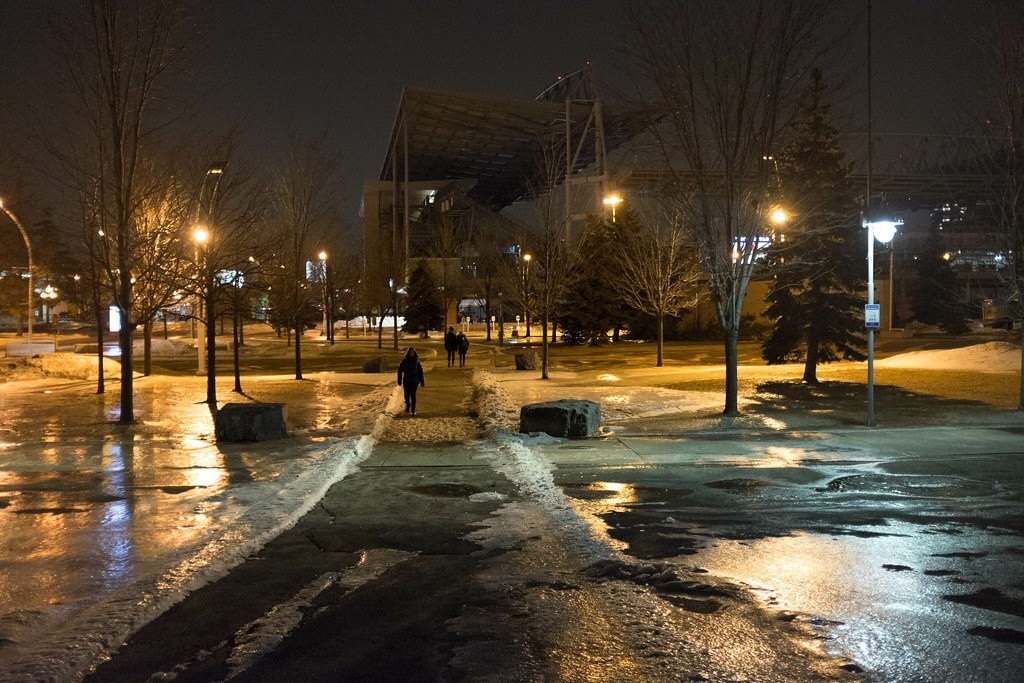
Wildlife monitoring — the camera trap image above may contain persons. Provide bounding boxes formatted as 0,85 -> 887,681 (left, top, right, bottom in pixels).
456,331 -> 469,367
397,347 -> 425,416
444,326 -> 457,367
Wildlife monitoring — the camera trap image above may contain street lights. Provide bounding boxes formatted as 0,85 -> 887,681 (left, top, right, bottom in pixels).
192,163 -> 225,375
604,192 -> 625,223
863,204 -> 905,425
